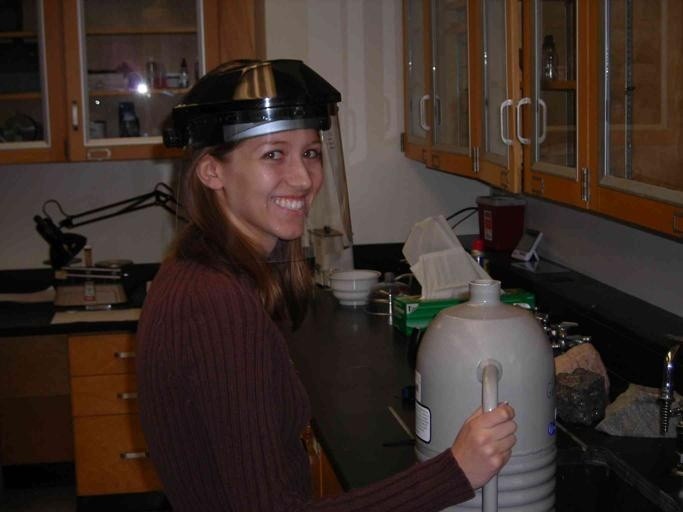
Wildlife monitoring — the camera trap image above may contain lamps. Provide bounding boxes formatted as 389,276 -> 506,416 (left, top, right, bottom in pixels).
31,180 -> 199,272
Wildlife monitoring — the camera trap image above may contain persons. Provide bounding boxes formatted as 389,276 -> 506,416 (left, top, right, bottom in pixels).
134,58 -> 516,511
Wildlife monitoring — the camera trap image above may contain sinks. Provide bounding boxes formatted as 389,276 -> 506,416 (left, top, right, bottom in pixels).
554,446 -> 683,512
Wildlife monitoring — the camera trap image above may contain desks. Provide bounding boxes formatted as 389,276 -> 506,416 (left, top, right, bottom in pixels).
1,267 -> 161,498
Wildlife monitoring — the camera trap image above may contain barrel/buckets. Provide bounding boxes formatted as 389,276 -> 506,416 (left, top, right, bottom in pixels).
413,278 -> 559,512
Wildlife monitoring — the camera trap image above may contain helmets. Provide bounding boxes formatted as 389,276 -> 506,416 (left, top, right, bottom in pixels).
164,57 -> 341,150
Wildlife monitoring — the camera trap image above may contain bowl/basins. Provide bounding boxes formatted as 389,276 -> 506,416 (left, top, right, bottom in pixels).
328,267 -> 381,306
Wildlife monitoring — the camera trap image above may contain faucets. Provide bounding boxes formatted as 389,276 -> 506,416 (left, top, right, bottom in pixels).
657,334 -> 683,476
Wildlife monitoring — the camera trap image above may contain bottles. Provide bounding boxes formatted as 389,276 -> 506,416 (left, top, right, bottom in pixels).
179,56 -> 189,88
542,34 -> 558,82
146,55 -> 156,90
470,237 -> 484,269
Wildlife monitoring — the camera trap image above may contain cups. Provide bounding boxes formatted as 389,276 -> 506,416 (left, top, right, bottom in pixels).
164,71 -> 179,88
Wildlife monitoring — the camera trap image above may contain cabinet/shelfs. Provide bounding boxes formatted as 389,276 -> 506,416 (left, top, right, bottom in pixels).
475,0 -> 586,212
0,1 -> 269,163
588,1 -> 683,243
309,442 -> 343,499
400,0 -> 476,181
